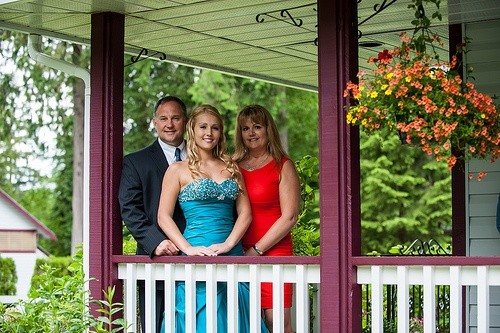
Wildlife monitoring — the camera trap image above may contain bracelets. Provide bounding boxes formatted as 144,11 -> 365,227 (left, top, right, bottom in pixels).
253,245 -> 264,255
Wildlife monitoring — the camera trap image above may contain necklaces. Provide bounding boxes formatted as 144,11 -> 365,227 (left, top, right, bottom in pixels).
244,152 -> 270,171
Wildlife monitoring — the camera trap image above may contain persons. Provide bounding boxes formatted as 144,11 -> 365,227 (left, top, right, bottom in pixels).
157,104 -> 269,333
230,104 -> 300,333
119,96 -> 188,333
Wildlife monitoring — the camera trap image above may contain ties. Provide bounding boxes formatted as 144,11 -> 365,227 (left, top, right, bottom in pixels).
175,148 -> 183,161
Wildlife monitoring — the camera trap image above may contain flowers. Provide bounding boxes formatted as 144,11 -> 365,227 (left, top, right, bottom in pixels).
341,0 -> 500,181
